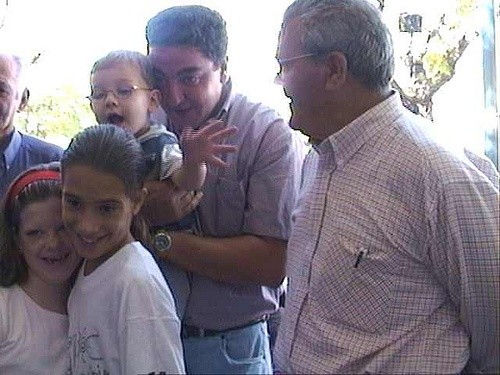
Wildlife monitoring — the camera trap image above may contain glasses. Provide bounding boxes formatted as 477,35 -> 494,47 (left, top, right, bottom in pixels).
85,83 -> 152,100
273,51 -> 316,74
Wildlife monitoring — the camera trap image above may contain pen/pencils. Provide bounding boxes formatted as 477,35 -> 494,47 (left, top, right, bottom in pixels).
353,247 -> 367,267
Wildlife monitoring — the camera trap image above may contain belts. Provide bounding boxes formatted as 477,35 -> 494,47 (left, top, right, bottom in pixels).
181,317 -> 262,336
